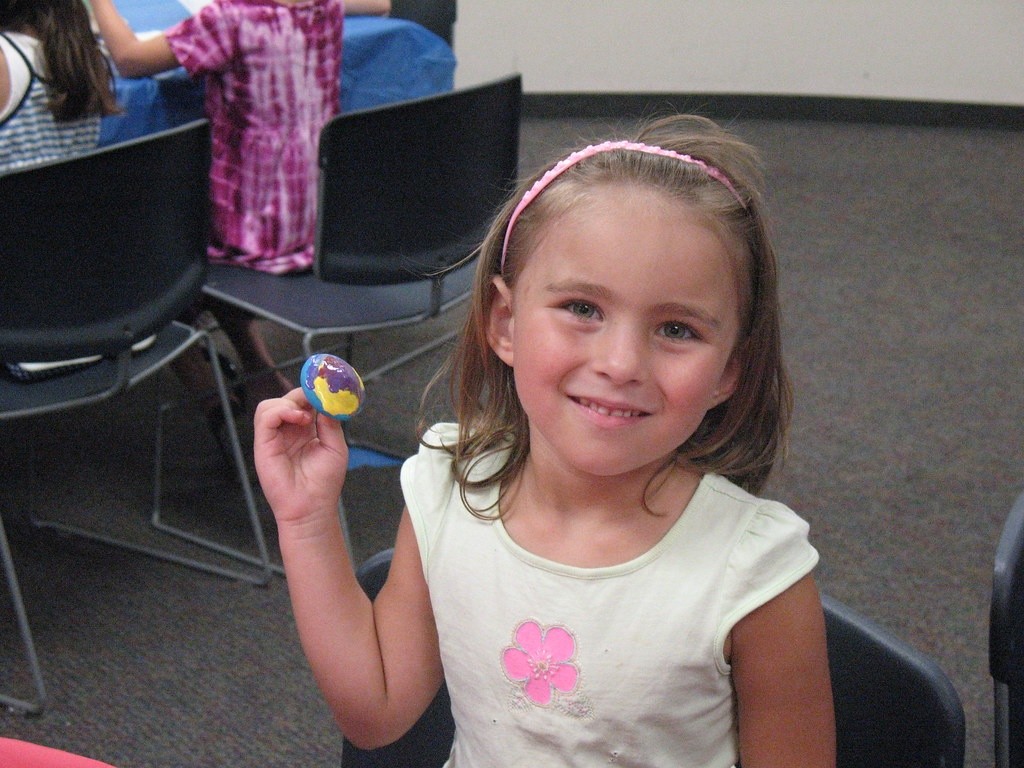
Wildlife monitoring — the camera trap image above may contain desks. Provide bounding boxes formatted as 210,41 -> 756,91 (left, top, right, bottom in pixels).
100,16 -> 456,144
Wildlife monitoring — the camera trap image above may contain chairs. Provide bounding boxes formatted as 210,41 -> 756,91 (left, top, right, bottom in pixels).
987,498 -> 1024,768
334,533 -> 966,768
148,72 -> 527,586
0,119 -> 274,718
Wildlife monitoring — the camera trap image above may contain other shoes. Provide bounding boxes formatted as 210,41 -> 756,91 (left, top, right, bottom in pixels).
205,390 -> 255,489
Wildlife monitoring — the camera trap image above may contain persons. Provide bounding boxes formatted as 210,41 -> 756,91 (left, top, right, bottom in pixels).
1,0 -> 161,386
251,113 -> 837,768
84,1 -> 391,462
